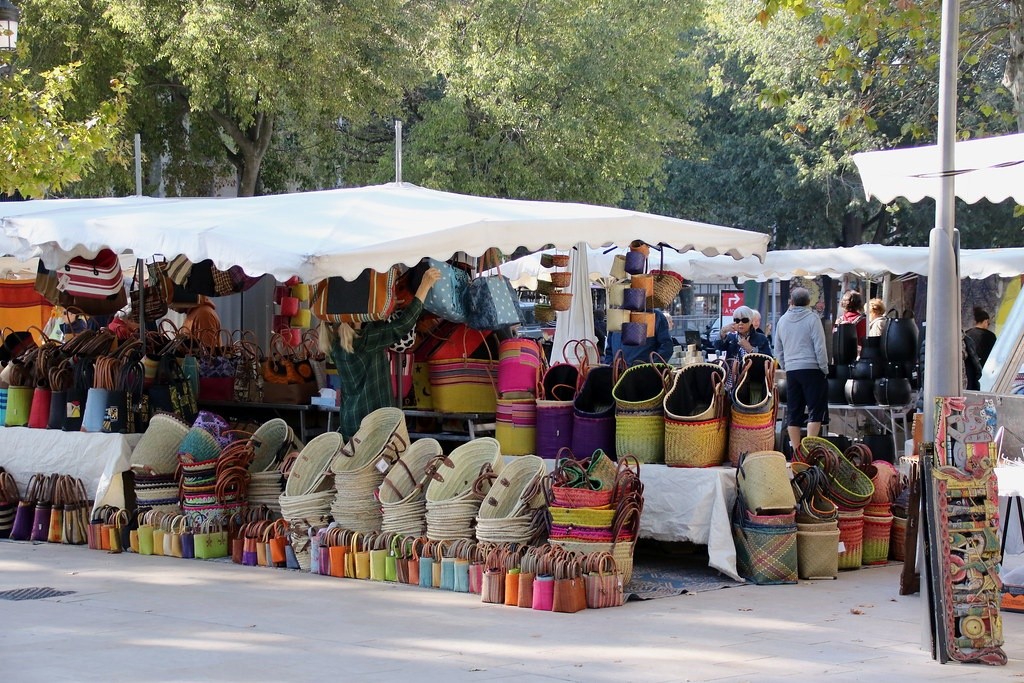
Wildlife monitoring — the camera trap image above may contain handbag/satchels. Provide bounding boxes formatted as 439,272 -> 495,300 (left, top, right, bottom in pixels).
0,240 -> 920,613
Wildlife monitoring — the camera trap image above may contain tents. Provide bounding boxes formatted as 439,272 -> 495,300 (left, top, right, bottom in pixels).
853,132 -> 1024,206
649,240 -> 1024,338
1,120 -> 772,285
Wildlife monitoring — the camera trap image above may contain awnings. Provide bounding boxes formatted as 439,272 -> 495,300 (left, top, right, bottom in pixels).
476,243 -> 730,337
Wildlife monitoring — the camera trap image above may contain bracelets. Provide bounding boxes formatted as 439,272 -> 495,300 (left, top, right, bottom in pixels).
749,349 -> 753,352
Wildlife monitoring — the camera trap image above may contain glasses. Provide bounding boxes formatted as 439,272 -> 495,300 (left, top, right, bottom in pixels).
734,318 -> 749,323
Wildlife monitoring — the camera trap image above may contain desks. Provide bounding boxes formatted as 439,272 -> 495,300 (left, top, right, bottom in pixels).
0,425 -> 144,518
226,401 -> 340,445
400,410 -> 496,441
503,455 -> 793,584
829,403 -> 909,463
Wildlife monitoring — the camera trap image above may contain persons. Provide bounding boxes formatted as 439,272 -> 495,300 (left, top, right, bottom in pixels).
663,313 -> 684,351
863,298 -> 887,337
605,309 -> 675,368
0,331 -> 39,389
774,287 -> 829,451
832,290 -> 866,384
318,267 -> 442,445
169,294 -> 222,346
965,306 -> 997,367
962,330 -> 983,391
713,306 -> 774,394
593,308 -> 607,335
108,298 -> 140,338
